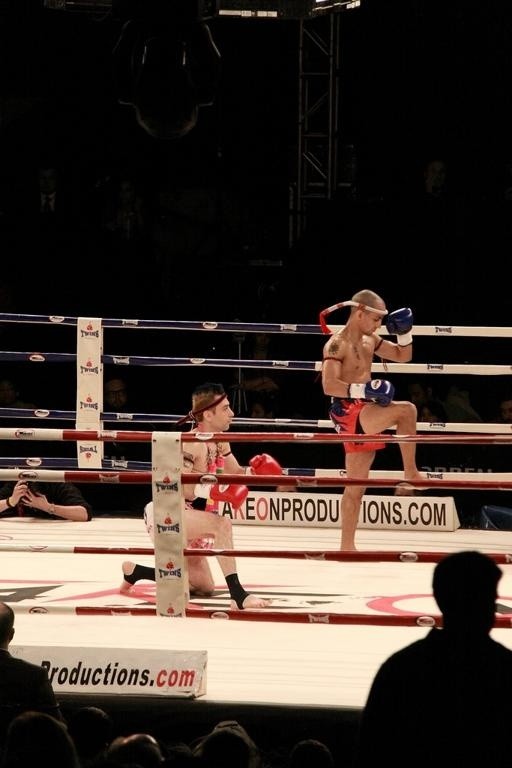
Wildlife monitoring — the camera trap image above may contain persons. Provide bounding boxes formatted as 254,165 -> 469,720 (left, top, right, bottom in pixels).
358,549 -> 510,768
0,708 -> 335,768
118,385 -> 287,611
319,289 -> 429,555
1,321 -> 511,529
1,600 -> 64,725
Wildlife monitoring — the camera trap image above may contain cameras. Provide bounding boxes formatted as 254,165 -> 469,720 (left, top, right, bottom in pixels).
21,480 -> 39,495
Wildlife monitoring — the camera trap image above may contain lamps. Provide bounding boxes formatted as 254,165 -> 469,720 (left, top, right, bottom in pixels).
107,0 -> 222,140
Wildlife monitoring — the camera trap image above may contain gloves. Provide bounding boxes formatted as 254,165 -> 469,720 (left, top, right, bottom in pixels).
195,483 -> 248,509
346,379 -> 395,406
386,308 -> 414,347
246,453 -> 282,476
